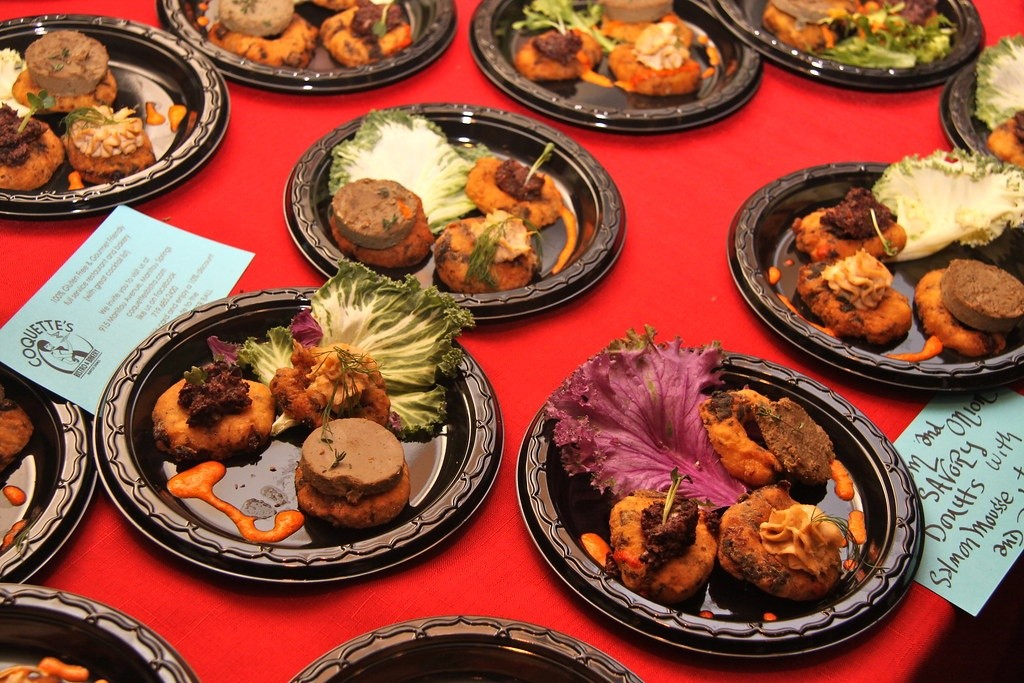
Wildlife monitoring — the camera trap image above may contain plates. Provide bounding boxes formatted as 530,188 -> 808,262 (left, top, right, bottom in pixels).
727,161 -> 1024,393
0,12 -> 230,220
92,286 -> 506,587
0,363 -> 100,585
156,0 -> 458,96
0,583 -> 202,683
288,614 -> 647,683
938,58 -> 1001,165
708,0 -> 986,92
514,347 -> 926,672
282,100 -> 627,323
467,0 -> 764,134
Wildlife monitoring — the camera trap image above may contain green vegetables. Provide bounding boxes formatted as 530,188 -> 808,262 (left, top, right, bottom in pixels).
19,0 -> 1024,513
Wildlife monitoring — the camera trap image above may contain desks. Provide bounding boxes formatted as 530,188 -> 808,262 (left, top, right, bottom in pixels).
0,0 -> 1024,683
